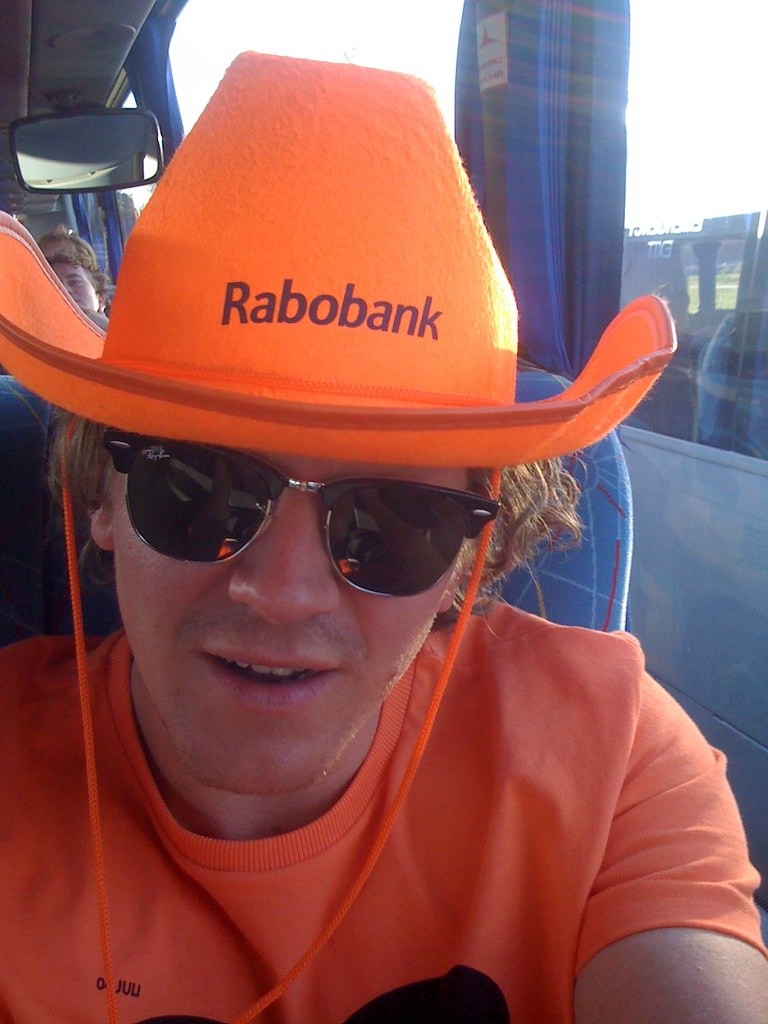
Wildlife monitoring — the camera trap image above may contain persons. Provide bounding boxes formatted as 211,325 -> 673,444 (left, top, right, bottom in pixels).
0,54 -> 768,1024
34,223 -> 112,329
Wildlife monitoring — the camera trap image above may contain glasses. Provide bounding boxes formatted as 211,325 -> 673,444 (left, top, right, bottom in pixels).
98,430 -> 505,598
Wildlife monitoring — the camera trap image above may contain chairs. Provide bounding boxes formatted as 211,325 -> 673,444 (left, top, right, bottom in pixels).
0,367 -> 635,635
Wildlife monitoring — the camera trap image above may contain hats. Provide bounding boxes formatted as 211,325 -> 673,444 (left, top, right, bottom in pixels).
0,47 -> 680,472
